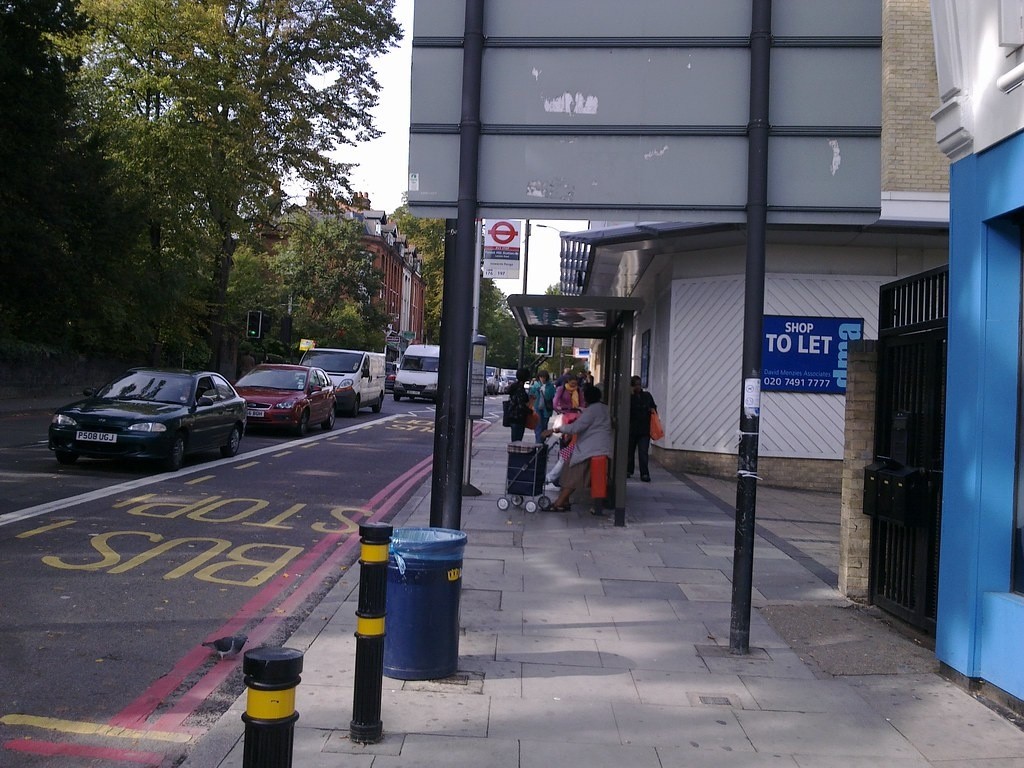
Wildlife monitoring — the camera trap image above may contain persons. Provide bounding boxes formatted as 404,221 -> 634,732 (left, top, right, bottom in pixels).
505,366 -> 659,512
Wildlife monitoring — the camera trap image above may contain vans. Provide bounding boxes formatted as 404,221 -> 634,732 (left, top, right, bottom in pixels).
298,347 -> 385,418
394,344 -> 440,403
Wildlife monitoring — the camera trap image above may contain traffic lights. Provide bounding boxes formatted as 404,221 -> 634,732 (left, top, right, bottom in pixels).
246,310 -> 262,339
535,337 -> 550,357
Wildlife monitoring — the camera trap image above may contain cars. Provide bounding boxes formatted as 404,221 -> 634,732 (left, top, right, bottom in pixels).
485,367 -> 517,395
386,362 -> 399,390
48,366 -> 247,471
233,363 -> 337,437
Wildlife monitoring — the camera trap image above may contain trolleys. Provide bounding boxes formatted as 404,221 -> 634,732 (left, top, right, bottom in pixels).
497,427 -> 560,513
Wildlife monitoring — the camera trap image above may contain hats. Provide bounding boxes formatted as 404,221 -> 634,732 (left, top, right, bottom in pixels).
631,375 -> 641,386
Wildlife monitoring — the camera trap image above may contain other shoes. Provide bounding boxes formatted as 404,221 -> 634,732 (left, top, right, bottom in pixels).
627,473 -> 631,478
541,502 -> 572,512
640,473 -> 651,482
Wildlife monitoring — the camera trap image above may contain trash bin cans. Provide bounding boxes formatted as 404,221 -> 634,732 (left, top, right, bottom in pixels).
377,526 -> 467,679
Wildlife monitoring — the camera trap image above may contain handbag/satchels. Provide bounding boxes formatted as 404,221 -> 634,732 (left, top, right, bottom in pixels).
544,399 -> 555,411
649,408 -> 665,441
503,401 -> 513,427
526,412 -> 539,429
